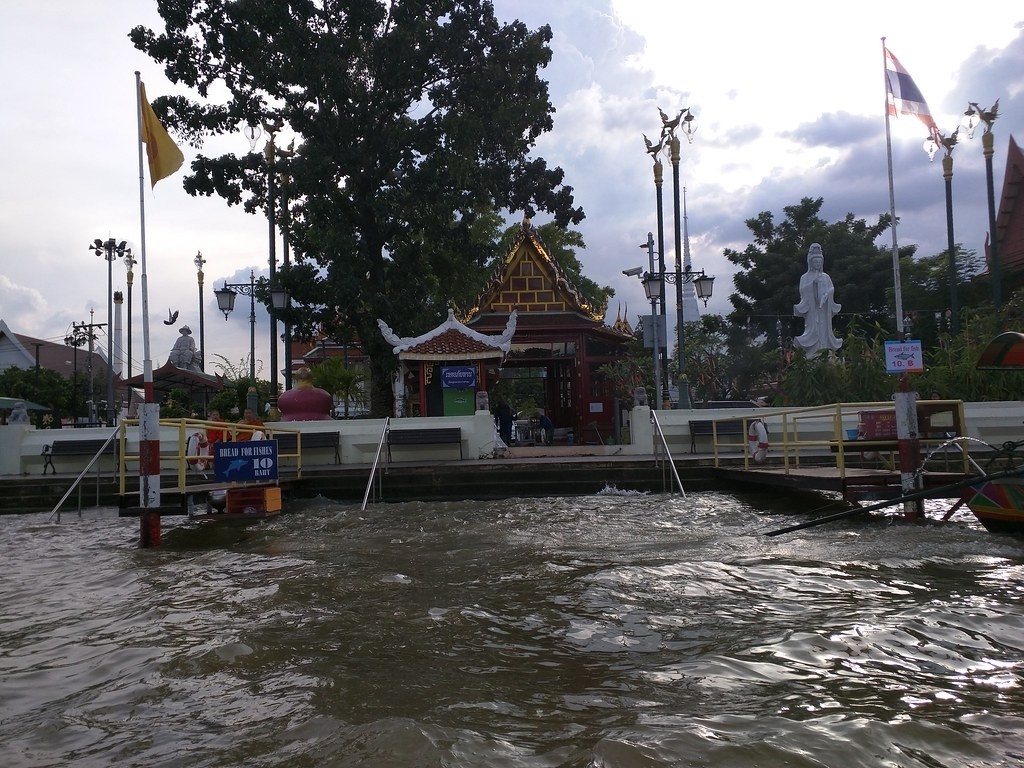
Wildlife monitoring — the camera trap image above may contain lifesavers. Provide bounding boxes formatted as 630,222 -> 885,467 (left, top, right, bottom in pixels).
188,431 -> 210,471
748,421 -> 769,463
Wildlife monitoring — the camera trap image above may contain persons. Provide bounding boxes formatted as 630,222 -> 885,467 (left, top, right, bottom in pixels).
206,409 -> 231,462
794,243 -> 843,360
169,325 -> 195,369
533,412 -> 554,446
494,400 -> 513,447
235,407 -> 266,442
926,392 -> 944,438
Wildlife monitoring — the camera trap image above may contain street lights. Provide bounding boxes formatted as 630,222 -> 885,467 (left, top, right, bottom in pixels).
88,236 -> 128,425
64,331 -> 88,427
123,248 -> 137,408
642,132 -> 673,409
962,101 -> 1004,310
214,282 -> 289,422
657,104 -> 717,409
31,343 -> 44,380
776,320 -> 783,359
924,124 -> 962,335
194,253 -> 206,373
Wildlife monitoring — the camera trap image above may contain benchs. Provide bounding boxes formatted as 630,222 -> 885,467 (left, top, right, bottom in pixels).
40,437 -> 128,476
688,418 -> 768,455
266,431 -> 342,465
386,427 -> 463,463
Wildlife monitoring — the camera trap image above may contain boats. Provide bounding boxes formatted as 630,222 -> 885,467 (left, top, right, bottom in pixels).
959,478 -> 1024,538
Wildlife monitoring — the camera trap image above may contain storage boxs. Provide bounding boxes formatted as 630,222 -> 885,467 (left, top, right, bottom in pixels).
226,486 -> 281,513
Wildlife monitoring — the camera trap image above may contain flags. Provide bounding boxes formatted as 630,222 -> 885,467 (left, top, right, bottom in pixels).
884,48 -> 940,149
140,82 -> 184,189
